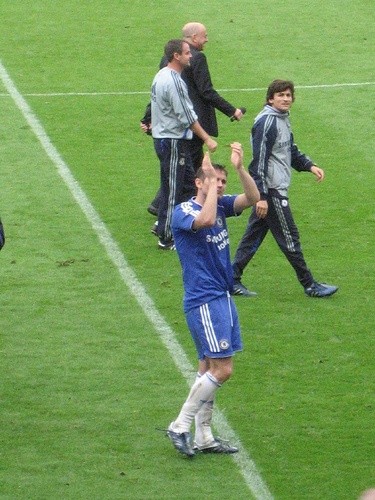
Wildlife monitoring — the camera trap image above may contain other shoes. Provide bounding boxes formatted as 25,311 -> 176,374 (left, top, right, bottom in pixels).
147,205 -> 158,216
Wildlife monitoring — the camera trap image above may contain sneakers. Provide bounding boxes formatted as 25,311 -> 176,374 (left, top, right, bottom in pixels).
151,224 -> 158,236
167,421 -> 195,456
305,281 -> 338,297
231,282 -> 257,296
192,436 -> 238,453
158,240 -> 177,251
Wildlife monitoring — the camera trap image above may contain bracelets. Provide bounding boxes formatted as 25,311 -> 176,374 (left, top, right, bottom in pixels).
312,163 -> 318,166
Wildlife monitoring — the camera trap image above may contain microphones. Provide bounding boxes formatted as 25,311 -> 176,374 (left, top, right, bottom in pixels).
230,108 -> 246,122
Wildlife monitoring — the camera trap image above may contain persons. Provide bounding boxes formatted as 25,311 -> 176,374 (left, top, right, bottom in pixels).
167,143 -> 261,458
141,22 -> 243,217
151,40 -> 218,250
231,79 -> 338,297
